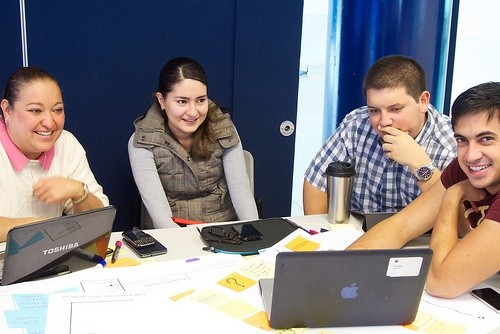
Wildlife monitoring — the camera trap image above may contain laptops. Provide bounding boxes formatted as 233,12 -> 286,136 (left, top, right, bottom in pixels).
0,205 -> 116,286
259,248 -> 434,329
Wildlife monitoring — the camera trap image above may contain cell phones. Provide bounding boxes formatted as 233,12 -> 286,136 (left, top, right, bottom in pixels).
122,230 -> 167,258
233,224 -> 263,242
470,288 -> 500,314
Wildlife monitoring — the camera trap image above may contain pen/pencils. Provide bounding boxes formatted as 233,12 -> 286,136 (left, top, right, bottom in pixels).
95,259 -> 107,267
73,250 -> 103,261
111,241 -> 123,263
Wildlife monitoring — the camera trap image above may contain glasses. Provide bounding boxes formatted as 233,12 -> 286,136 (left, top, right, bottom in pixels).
196,225 -> 242,245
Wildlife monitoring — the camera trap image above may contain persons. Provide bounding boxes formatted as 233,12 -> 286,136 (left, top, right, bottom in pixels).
0,67 -> 109,243
345,82 -> 500,299
303,55 -> 457,234
128,57 -> 259,230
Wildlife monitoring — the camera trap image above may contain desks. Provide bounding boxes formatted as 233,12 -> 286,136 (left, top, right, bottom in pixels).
0,212 -> 500,334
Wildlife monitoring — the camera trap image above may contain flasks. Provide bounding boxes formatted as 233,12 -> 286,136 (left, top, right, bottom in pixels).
324,161 -> 357,225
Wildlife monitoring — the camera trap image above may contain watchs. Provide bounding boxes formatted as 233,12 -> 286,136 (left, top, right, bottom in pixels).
412,162 -> 435,181
71,182 -> 89,203
